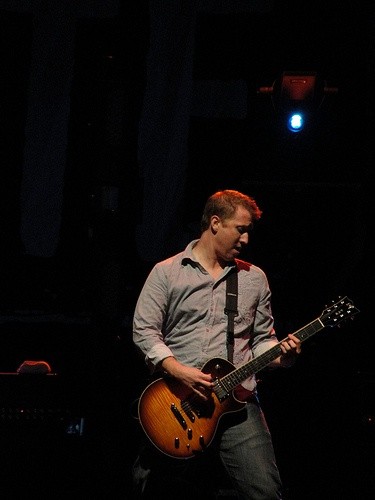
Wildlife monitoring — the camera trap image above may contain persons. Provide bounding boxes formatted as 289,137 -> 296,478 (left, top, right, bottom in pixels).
128,190 -> 304,500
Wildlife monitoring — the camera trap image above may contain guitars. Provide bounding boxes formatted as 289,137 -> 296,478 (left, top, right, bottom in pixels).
136,294 -> 362,462
